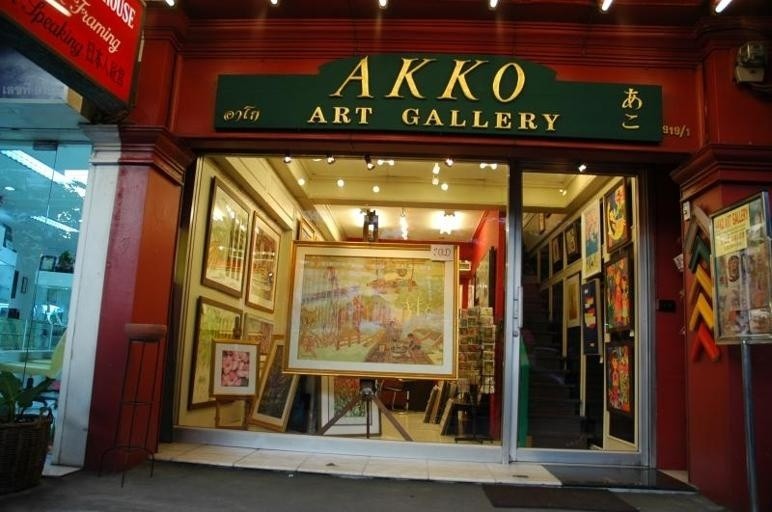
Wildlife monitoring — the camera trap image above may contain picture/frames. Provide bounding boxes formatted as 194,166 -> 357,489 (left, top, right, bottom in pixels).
580,199 -> 604,280
187,295 -> 243,411
604,339 -> 633,419
281,240 -> 459,381
563,222 -> 579,262
243,311 -> 277,393
199,175 -> 252,298
580,281 -> 599,354
245,208 -> 283,314
601,250 -> 633,334
246,334 -> 301,431
565,271 -> 582,330
601,179 -> 631,252
209,337 -> 260,400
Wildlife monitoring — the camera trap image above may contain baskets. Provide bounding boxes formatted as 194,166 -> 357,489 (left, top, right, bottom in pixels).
0,407 -> 54,494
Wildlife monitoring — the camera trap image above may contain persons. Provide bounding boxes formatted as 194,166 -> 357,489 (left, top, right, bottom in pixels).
388,322 -> 397,343
408,333 -> 422,351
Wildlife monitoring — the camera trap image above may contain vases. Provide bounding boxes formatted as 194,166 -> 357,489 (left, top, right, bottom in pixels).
319,376 -> 383,437
